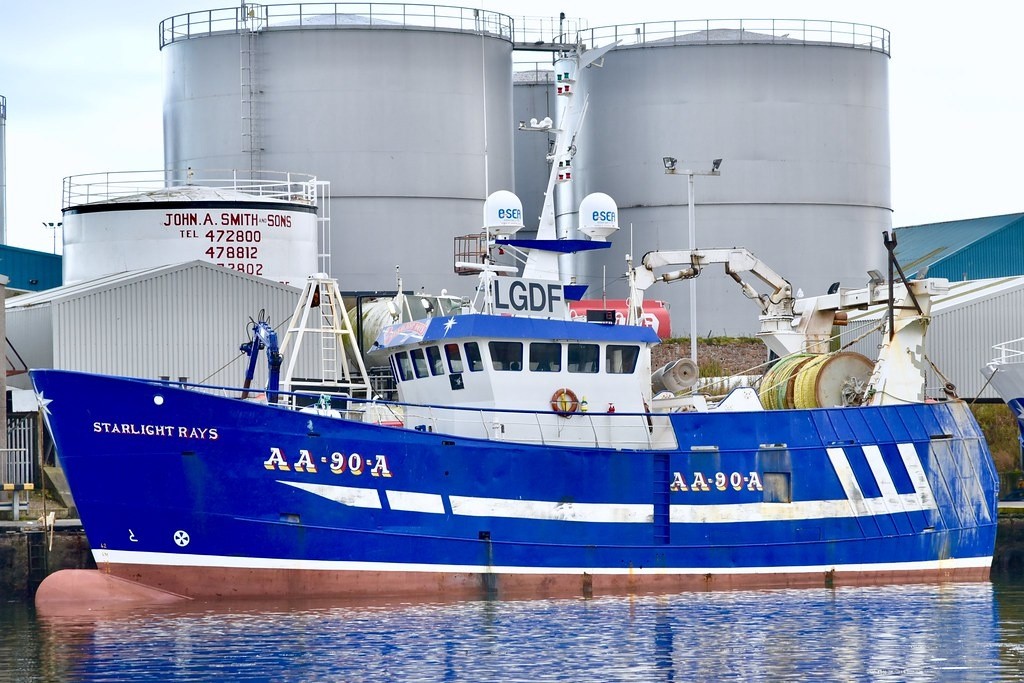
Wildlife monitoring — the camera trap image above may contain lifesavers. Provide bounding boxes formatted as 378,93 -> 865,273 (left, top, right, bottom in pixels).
551,388 -> 578,417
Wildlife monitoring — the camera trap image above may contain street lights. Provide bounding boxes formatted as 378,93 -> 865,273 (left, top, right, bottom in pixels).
662,156 -> 726,394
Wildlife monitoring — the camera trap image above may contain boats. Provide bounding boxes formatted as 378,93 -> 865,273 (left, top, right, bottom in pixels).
26,37 -> 1000,624
978,335 -> 1024,510
